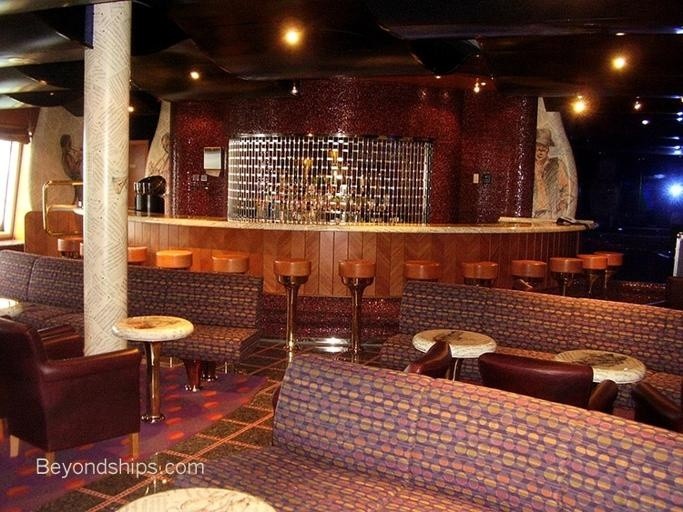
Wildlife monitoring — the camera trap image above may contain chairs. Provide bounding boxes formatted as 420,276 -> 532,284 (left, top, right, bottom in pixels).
0,316 -> 143,476
479,352 -> 617,415
631,383 -> 683,433
405,342 -> 451,378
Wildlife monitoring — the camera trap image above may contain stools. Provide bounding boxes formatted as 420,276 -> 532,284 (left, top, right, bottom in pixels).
403,251 -> 623,295
58,236 -> 249,277
275,259 -> 311,353
339,260 -> 375,357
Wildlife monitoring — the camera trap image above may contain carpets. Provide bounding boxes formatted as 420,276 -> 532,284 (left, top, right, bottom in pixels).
34,340 -> 380,512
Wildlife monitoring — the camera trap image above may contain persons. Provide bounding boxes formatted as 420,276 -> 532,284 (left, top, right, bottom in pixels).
532,128 -> 569,219
59,134 -> 83,204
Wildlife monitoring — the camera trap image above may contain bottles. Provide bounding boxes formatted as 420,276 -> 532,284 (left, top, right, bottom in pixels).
235,150 -> 404,223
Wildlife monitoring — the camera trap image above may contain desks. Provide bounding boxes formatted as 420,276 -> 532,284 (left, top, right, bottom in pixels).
413,330 -> 497,382
112,315 -> 194,424
556,350 -> 645,384
0,298 -> 23,319
117,488 -> 277,512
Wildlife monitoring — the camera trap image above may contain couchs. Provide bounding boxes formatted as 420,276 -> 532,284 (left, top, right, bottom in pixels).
380,280 -> 683,411
175,355 -> 682,512
0,247 -> 264,392
